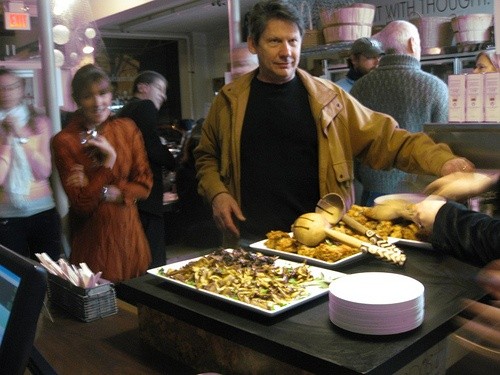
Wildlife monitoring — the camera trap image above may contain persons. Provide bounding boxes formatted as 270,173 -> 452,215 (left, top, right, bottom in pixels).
117,71 -> 181,269
409,195 -> 500,266
349,20 -> 451,206
196,1 -> 474,244
474,50 -> 500,73
335,38 -> 384,94
51,64 -> 153,296
0,65 -> 64,300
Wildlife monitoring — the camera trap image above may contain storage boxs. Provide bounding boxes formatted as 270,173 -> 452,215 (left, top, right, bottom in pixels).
47,261 -> 119,322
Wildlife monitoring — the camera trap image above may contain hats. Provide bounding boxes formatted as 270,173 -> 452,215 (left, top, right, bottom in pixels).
351,37 -> 386,57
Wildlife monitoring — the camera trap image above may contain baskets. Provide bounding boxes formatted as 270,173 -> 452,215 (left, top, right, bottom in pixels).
301,0 -> 323,48
408,11 -> 456,48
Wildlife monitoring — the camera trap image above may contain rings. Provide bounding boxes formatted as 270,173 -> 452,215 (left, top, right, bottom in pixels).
462,166 -> 466,170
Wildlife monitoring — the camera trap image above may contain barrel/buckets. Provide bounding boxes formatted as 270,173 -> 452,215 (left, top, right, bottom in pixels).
409,16 -> 455,55
451,13 -> 493,51
319,4 -> 376,42
232,43 -> 259,75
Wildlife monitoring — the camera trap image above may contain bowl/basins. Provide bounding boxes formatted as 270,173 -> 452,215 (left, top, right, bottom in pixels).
373,193 -> 446,207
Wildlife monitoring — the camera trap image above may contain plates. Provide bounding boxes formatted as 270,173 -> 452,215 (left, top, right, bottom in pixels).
385,235 -> 435,248
328,272 -> 425,334
249,233 -> 368,271
146,248 -> 347,317
